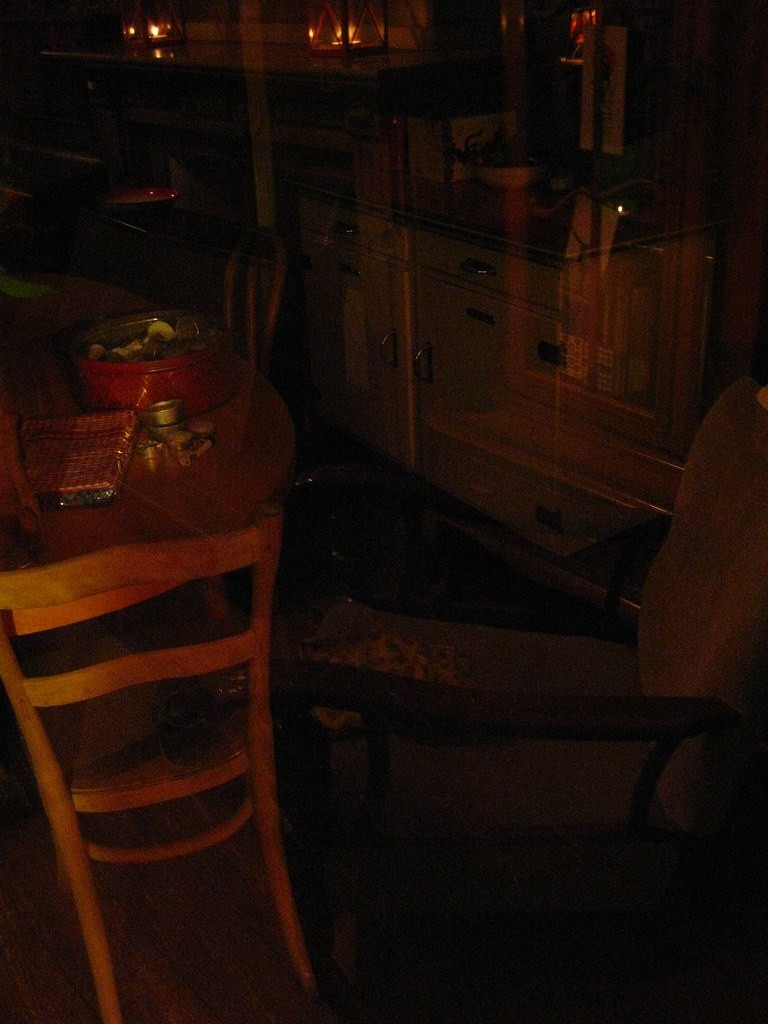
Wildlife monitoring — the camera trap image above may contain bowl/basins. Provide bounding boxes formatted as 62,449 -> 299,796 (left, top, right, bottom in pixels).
468,160 -> 545,189
66,310 -> 239,423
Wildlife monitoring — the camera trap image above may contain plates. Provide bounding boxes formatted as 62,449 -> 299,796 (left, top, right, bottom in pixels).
97,187 -> 179,211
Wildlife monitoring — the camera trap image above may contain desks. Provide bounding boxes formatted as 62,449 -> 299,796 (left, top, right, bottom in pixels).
0,270 -> 296,821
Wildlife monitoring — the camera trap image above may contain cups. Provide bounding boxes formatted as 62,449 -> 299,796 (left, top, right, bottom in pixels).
145,398 -> 183,442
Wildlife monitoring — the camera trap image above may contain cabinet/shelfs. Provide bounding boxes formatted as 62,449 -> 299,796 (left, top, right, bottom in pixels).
296,176 -> 728,557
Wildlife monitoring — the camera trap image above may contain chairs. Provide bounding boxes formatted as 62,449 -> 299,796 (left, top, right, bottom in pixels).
0,501 -> 317,1024
283,375 -> 768,1004
